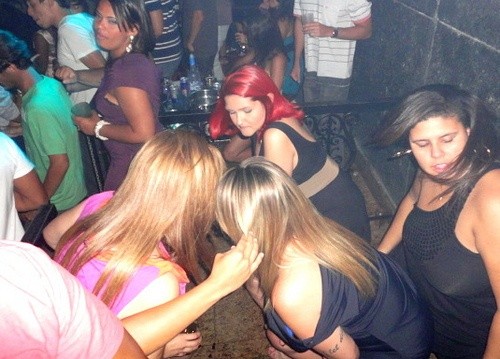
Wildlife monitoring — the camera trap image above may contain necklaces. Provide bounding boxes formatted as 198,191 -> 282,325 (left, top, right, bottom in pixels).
439,183 -> 448,200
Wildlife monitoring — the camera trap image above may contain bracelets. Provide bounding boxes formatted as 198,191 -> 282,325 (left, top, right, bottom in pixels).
94,120 -> 112,141
331,26 -> 339,38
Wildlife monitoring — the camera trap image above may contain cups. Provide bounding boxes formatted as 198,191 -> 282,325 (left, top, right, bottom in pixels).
71,102 -> 92,118
205,74 -> 214,88
301,13 -> 314,25
180,321 -> 196,333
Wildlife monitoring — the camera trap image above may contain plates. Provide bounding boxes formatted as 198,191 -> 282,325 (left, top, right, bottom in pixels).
191,88 -> 219,107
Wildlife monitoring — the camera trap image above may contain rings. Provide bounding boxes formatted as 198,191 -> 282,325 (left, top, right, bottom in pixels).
77,125 -> 81,130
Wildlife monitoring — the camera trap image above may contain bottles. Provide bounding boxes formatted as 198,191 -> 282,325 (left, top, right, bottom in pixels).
187,54 -> 201,83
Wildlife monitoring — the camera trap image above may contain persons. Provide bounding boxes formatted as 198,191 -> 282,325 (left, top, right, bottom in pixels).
214,156 -> 435,359
0,0 -> 219,135
0,30 -> 98,217
208,64 -> 372,242
366,84 -> 500,359
55,0 -> 165,192
43,129 -> 229,359
1,232 -> 264,359
291,0 -> 373,166
0,131 -> 48,241
219,0 -> 304,103
